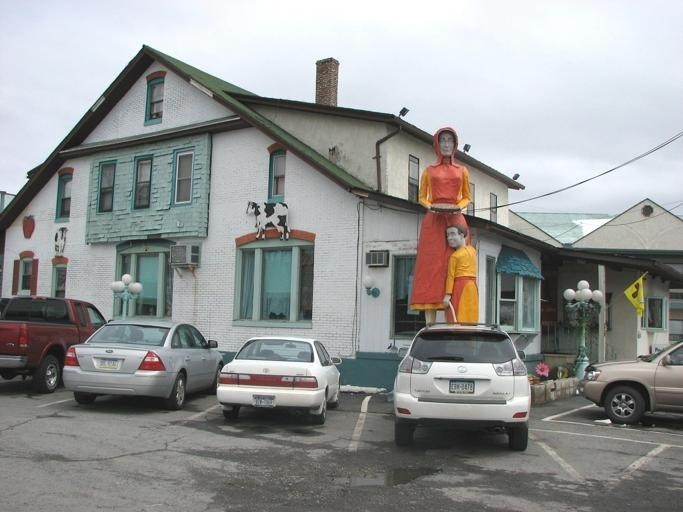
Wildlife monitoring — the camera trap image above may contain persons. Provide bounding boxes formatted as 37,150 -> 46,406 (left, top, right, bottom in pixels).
410,127 -> 472,326
443,224 -> 479,324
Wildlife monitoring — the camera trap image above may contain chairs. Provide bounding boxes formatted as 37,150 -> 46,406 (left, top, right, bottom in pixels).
259,350 -> 312,360
477,343 -> 497,362
125,329 -> 144,344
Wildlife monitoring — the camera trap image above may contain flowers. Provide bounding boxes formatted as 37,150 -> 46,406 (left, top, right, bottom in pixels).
535,363 -> 550,382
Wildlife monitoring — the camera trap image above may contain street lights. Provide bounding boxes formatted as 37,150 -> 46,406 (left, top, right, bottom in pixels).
564,280 -> 603,379
111,274 -> 142,319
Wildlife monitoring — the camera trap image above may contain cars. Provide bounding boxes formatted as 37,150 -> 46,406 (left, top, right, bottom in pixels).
393,322 -> 532,451
216,336 -> 342,426
62,316 -> 224,411
585,340 -> 683,424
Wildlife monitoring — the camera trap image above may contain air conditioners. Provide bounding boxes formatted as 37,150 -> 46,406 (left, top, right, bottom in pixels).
169,243 -> 200,265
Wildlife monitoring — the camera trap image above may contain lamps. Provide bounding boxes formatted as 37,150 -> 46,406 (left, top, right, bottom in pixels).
361,275 -> 382,297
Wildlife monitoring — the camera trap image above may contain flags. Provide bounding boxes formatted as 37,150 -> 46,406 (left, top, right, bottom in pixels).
624,276 -> 645,317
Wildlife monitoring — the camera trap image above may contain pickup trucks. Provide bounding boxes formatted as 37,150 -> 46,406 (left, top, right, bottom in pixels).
0,296 -> 120,394
